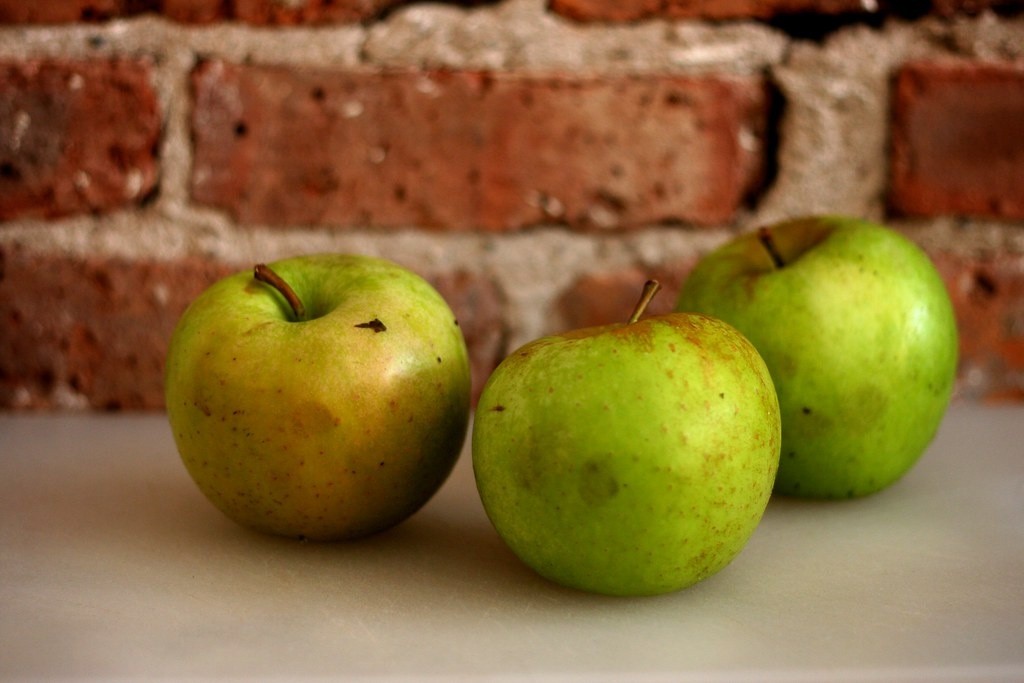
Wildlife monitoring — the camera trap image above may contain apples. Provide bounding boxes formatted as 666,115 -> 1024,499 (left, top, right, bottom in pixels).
677,212 -> 960,499
160,247 -> 472,543
471,277 -> 784,596
